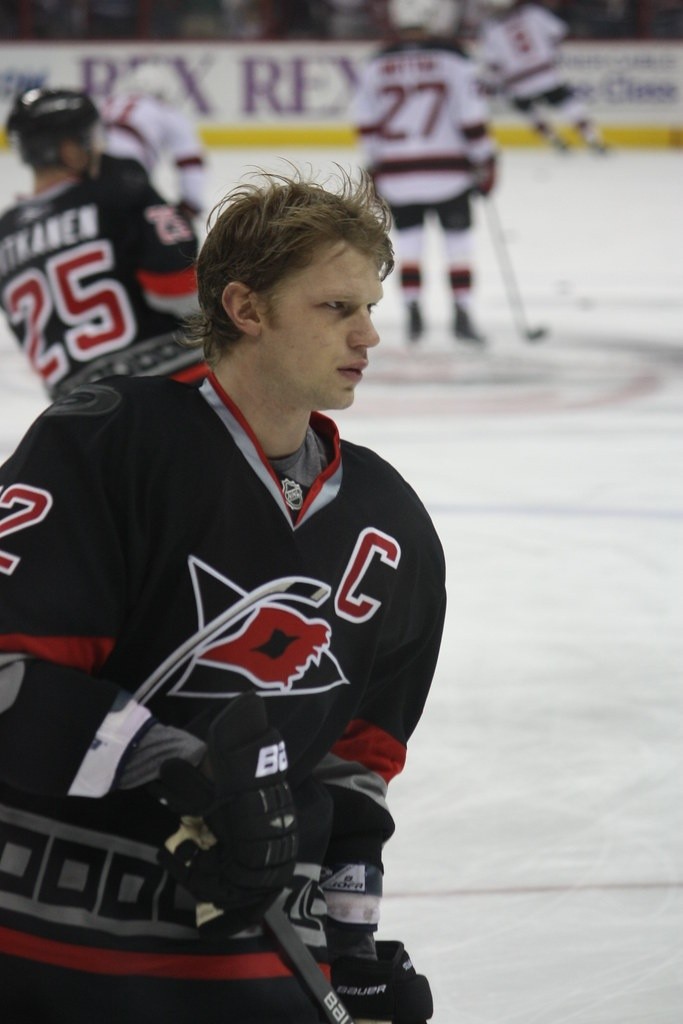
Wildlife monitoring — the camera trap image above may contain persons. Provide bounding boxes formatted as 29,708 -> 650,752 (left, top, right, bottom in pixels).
0,0 -> 616,402
0,165 -> 446,1024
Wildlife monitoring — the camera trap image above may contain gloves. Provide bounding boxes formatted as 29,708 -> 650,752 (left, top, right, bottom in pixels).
156,687 -> 299,937
332,940 -> 433,1024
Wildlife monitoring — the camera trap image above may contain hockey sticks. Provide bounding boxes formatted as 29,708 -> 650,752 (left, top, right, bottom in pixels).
483,192 -> 545,342
260,900 -> 356,1024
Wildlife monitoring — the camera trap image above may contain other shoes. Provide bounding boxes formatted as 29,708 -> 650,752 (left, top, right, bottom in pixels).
543,129 -> 573,155
449,308 -> 483,343
576,125 -> 613,154
405,296 -> 424,341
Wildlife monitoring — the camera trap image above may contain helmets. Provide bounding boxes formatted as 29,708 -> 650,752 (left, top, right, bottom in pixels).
122,62 -> 187,110
15,87 -> 99,162
388,0 -> 464,40
